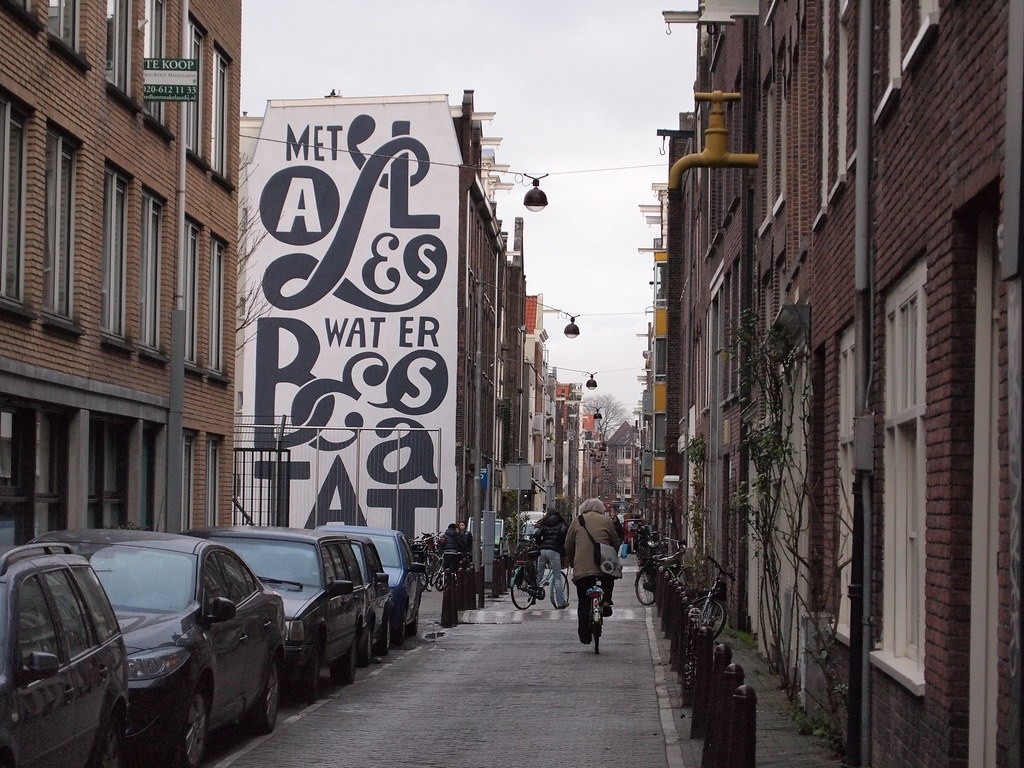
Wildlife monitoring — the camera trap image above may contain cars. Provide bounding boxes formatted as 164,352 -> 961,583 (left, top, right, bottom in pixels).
328,531 -> 393,666
22,529 -> 287,768
182,524 -> 372,707
314,523 -> 426,649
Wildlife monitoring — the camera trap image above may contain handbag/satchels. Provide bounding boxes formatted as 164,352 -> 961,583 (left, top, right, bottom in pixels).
594,543 -> 622,579
645,560 -> 657,577
603,602 -> 612,617
524,560 -> 536,585
618,543 -> 628,559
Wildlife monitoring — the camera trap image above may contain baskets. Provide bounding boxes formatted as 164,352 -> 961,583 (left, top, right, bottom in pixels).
560,547 -> 569,569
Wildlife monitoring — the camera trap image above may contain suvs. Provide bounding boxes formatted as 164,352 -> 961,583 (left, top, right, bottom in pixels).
0,542 -> 129,768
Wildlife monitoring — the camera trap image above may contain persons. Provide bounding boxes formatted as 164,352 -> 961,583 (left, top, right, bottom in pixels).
436,507 -> 642,609
564,499 -> 624,645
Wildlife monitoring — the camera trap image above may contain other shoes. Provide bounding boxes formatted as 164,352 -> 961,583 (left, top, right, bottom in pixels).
558,601 -> 569,608
528,597 -> 535,604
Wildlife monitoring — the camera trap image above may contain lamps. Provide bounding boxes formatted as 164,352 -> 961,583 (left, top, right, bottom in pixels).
520,174 -> 548,212
586,372 -> 597,390
564,312 -> 580,339
594,407 -> 602,420
599,442 -> 607,452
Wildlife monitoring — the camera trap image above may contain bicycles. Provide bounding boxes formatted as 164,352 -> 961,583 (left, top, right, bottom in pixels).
587,577 -> 608,654
692,556 -> 736,639
624,517 -> 710,604
509,549 -> 570,611
406,531 -> 452,592
